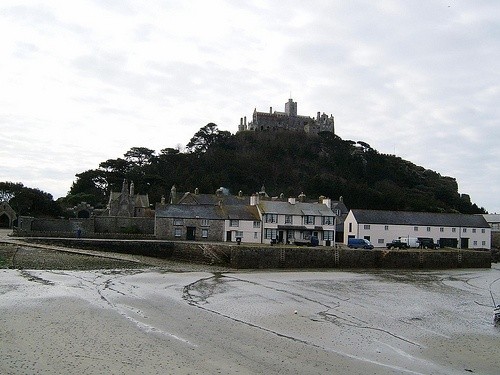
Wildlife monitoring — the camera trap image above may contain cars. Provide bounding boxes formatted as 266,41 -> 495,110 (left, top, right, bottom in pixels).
418,239 -> 434,249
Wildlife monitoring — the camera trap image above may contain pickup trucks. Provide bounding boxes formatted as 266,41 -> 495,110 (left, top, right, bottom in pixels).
385,239 -> 407,248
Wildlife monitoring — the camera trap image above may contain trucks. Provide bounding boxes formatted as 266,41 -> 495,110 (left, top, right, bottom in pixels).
294,235 -> 320,247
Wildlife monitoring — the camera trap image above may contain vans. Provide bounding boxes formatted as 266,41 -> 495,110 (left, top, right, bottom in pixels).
348,238 -> 374,249
